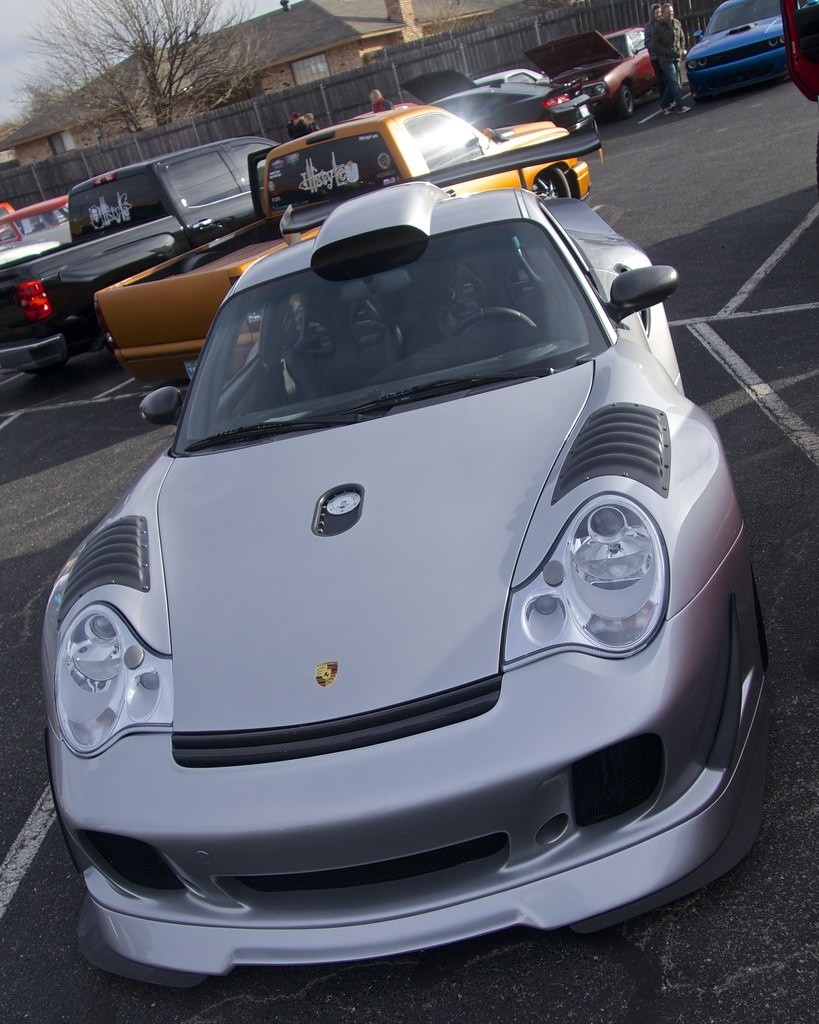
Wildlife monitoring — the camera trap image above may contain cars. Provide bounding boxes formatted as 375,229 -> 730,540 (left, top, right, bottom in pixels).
780,0 -> 819,105
0,192 -> 70,261
685,0 -> 819,101
336,26 -> 657,124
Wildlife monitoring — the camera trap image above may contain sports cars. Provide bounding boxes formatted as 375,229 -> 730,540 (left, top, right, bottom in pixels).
38,179 -> 775,989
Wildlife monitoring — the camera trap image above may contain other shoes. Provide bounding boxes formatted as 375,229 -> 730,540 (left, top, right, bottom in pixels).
669,103 -> 676,110
678,106 -> 691,113
661,106 -> 671,115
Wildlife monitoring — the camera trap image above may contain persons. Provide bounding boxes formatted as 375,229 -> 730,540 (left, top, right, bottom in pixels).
644,4 -> 675,109
369,89 -> 392,113
287,111 -> 321,140
650,2 -> 691,115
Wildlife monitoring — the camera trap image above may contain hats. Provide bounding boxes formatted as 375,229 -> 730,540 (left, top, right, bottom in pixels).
288,112 -> 300,121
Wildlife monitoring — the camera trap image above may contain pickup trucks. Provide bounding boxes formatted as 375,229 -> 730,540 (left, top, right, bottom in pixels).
93,104 -> 591,380
1,137 -> 278,380
